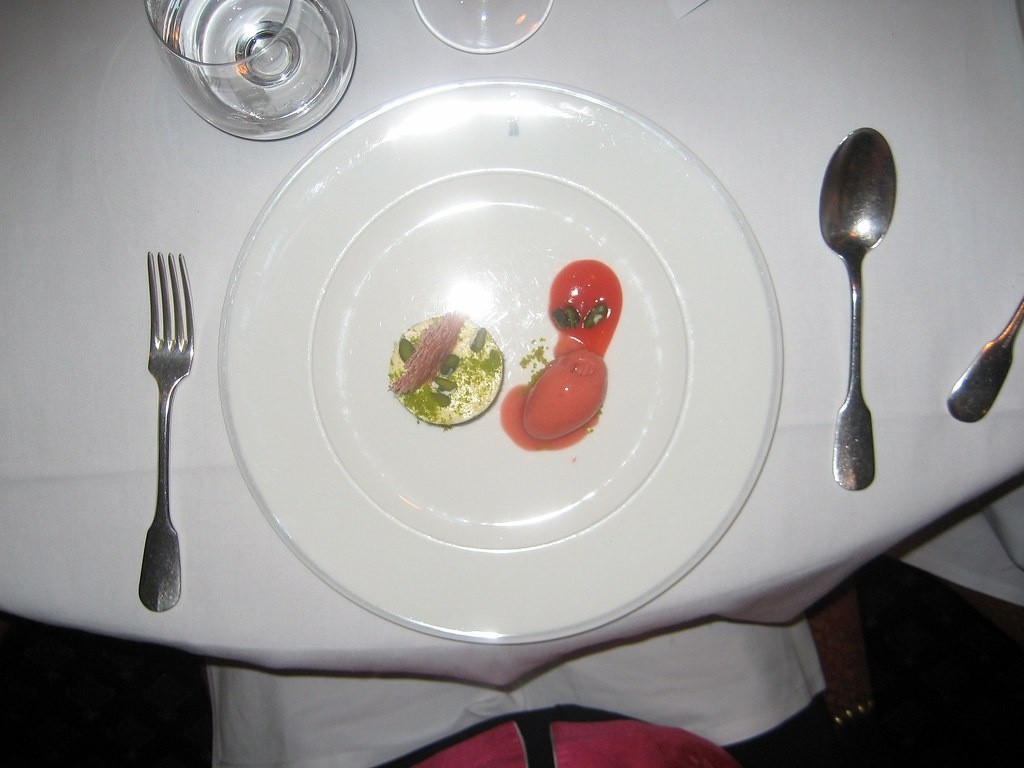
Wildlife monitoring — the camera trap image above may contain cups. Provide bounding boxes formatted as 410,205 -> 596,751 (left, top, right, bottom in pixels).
142,1 -> 357,146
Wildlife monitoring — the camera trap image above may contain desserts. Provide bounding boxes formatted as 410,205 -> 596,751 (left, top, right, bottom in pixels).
522,349 -> 609,440
389,313 -> 505,428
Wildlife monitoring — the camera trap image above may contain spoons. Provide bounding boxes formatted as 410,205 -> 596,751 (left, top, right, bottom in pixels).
820,126 -> 898,490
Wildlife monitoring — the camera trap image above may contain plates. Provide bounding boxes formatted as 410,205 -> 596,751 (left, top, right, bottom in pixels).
215,75 -> 784,646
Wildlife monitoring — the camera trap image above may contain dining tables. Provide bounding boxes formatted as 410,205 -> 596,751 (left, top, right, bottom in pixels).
1,0 -> 1022,691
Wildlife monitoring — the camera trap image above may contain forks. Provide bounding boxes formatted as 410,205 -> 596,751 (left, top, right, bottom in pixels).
139,251 -> 194,612
